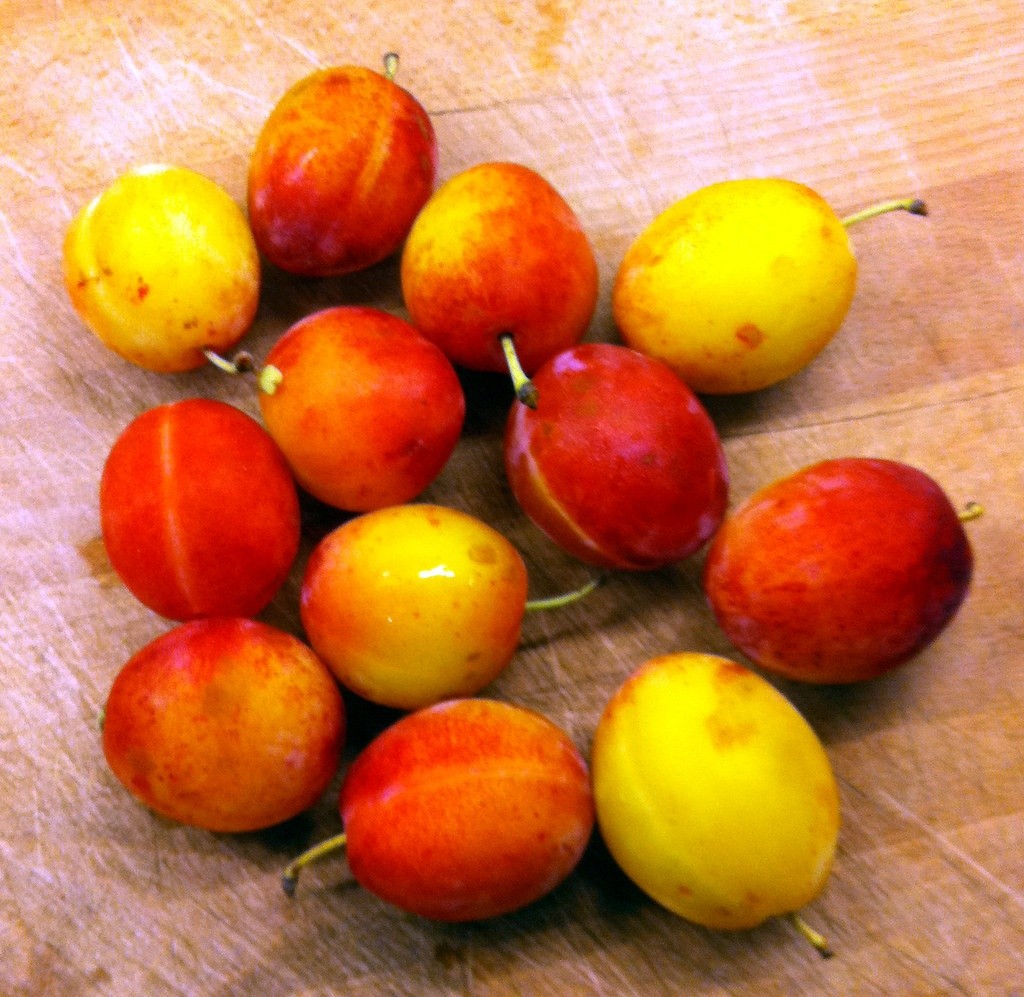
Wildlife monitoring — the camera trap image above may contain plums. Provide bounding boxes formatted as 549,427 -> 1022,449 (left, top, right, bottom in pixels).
62,52 -> 981,960
